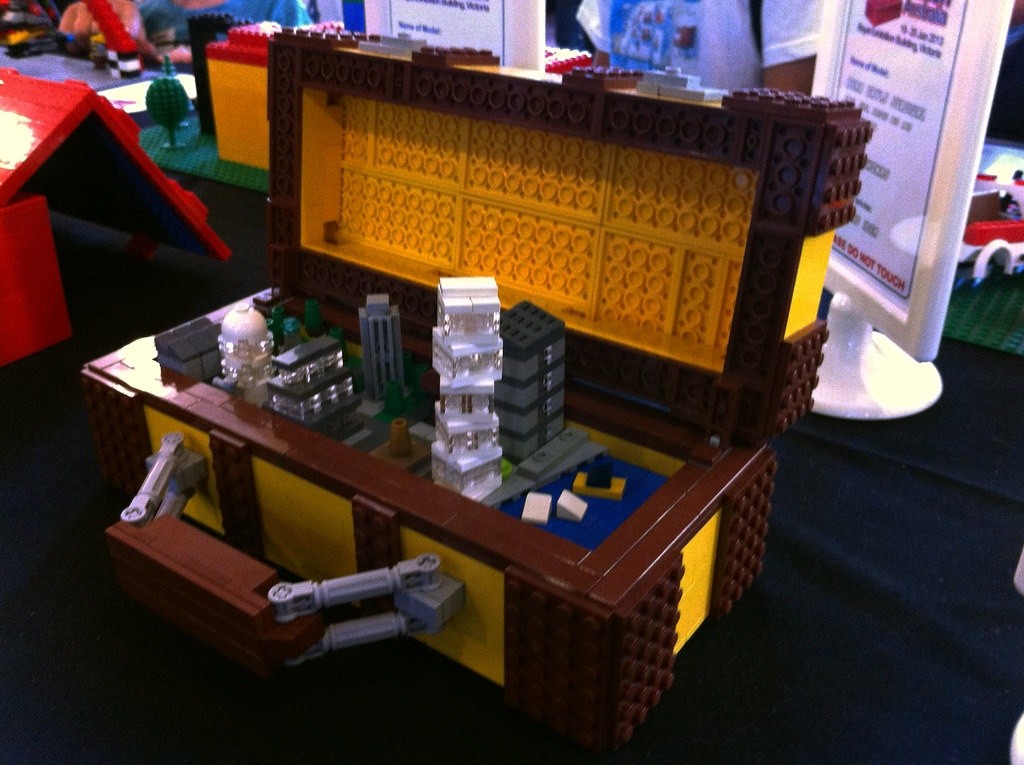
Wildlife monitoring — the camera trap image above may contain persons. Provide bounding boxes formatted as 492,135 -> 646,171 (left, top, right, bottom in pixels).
59,0 -> 312,67
